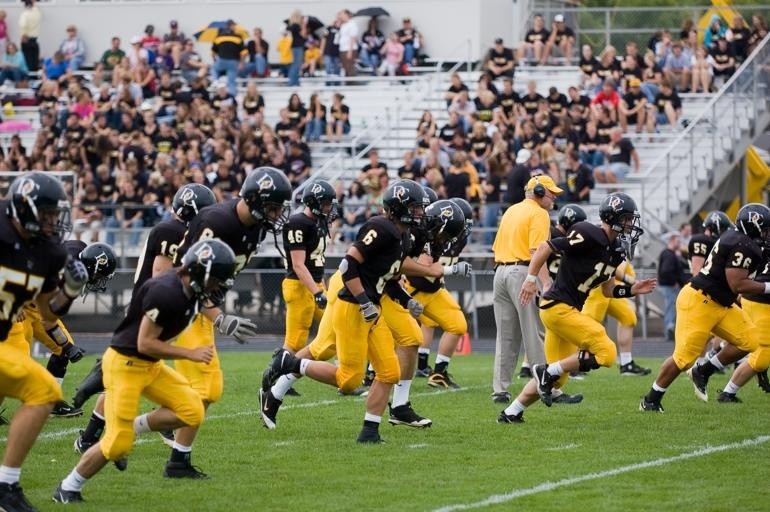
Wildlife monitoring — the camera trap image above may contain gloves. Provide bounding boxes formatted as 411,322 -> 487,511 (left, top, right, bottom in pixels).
359,302 -> 378,322
408,299 -> 424,318
214,313 -> 257,344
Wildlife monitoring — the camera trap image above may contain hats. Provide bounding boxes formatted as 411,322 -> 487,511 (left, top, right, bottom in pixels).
524,174 -> 564,196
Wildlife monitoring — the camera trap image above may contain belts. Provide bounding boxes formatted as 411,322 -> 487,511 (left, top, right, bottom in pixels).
500,260 -> 530,268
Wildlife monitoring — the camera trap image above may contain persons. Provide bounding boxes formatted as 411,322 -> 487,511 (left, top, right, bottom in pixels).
493,176 -> 657,423
262,178 -> 476,441
0,165 -> 294,512
3,0 -> 422,245
326,12 -> 769,244
640,202 -> 770,412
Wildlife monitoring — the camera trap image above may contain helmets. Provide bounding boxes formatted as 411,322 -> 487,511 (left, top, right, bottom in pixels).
557,193 -> 770,260
